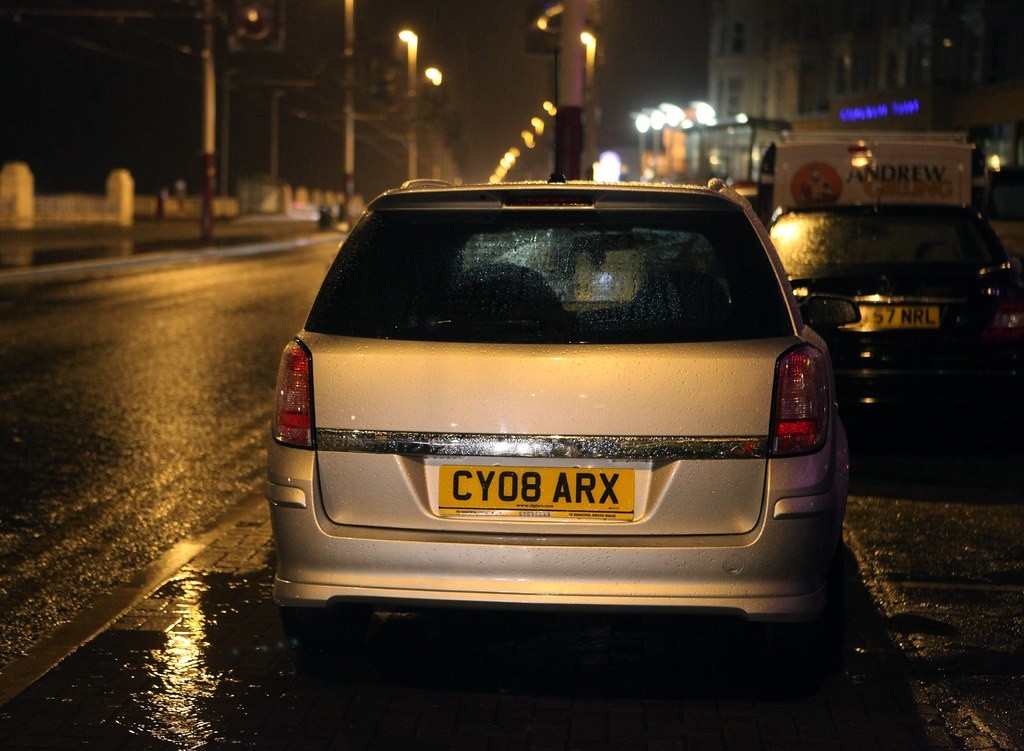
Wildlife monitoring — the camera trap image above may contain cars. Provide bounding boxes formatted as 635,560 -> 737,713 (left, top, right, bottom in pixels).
763,201 -> 1024,467
260,175 -> 860,681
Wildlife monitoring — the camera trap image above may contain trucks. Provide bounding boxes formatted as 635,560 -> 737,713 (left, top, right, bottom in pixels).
728,133 -> 986,215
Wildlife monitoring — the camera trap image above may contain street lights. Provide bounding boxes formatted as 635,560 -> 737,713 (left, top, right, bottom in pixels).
398,28 -> 419,179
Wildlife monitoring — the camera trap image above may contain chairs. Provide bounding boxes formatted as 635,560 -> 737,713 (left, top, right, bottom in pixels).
631,264 -> 730,325
449,263 -> 570,339
914,240 -> 947,262
